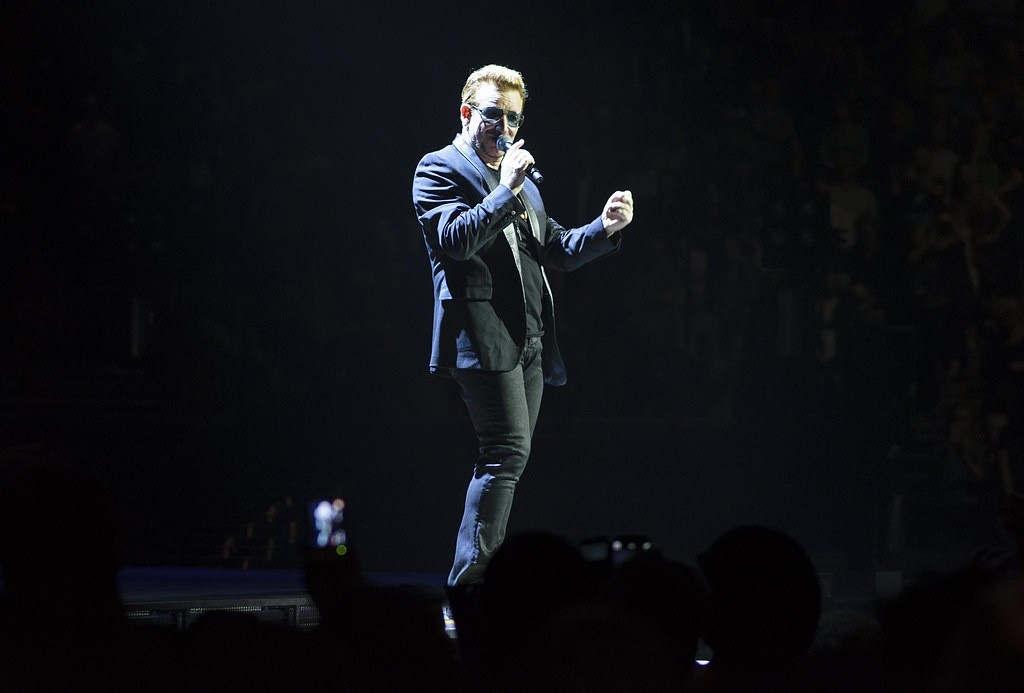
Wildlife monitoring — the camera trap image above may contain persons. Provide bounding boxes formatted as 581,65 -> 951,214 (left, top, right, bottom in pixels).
411,64 -> 635,641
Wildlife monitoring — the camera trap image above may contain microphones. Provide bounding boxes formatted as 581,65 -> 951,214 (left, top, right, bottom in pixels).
496,135 -> 543,183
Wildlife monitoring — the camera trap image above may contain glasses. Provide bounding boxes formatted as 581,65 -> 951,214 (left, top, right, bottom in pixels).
473,105 -> 524,127
